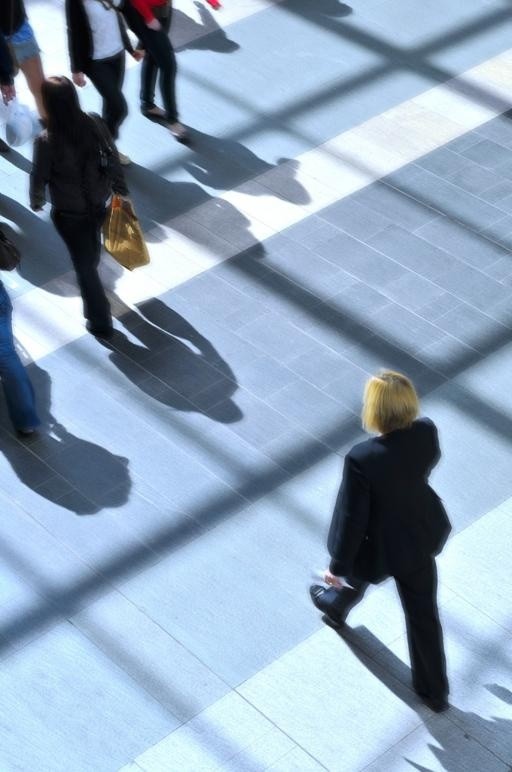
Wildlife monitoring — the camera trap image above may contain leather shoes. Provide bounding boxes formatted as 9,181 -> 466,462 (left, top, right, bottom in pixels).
141,104 -> 192,143
421,691 -> 449,712
310,585 -> 344,627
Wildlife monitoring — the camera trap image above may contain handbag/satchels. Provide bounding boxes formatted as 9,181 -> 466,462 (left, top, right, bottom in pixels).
102,193 -> 149,270
0,230 -> 20,270
0,94 -> 33,147
120,2 -> 171,40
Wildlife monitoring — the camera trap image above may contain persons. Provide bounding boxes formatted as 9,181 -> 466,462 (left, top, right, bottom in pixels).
0,281 -> 41,436
121,1 -> 186,139
309,370 -> 451,712
65,0 -> 139,167
1,34 -> 17,154
29,77 -> 129,338
0,0 -> 46,128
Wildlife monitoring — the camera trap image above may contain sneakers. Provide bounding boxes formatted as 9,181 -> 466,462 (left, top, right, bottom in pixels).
85,319 -> 113,339
